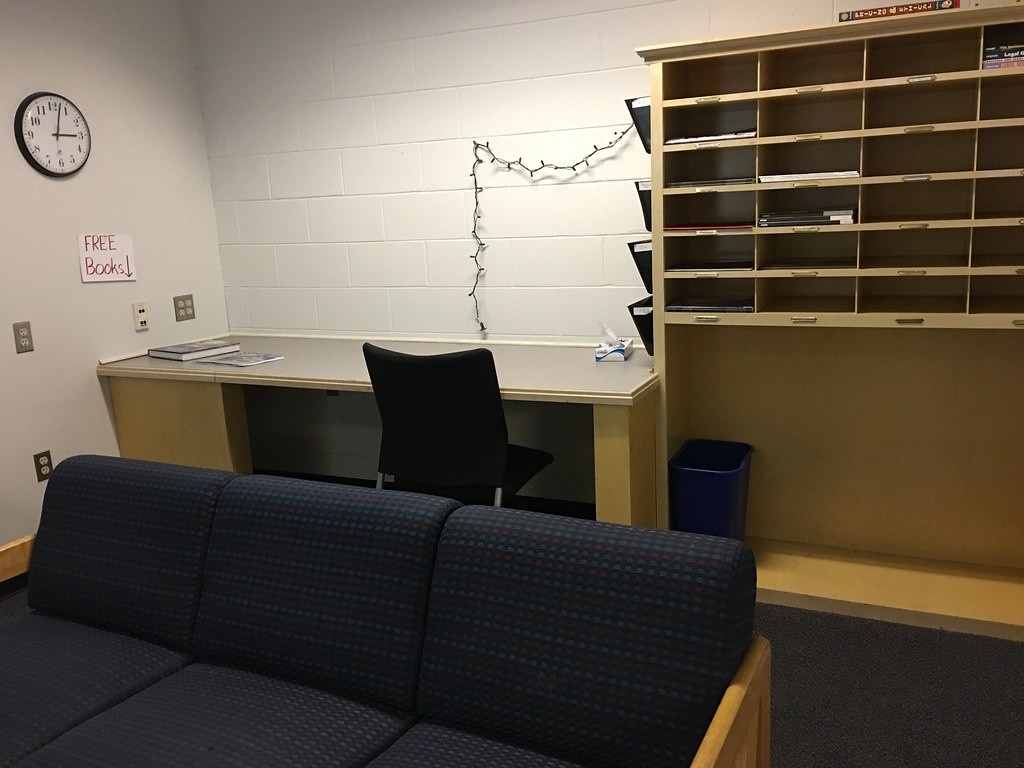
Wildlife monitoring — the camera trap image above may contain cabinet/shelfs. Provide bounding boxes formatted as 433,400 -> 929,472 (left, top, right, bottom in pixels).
637,4 -> 1024,642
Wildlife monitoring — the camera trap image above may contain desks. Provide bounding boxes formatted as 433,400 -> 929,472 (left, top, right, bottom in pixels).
96,335 -> 658,528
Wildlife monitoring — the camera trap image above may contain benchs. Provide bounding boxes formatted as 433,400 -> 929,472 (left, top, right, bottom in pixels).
0,453 -> 771,768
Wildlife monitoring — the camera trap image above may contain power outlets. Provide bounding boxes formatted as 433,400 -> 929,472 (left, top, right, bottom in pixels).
33,450 -> 53,483
173,294 -> 195,322
12,321 -> 34,354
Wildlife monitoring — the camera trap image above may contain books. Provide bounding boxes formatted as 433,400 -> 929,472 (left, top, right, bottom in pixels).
664,222 -> 753,229
758,207 -> 853,227
208,351 -> 285,366
983,44 -> 1024,69
667,261 -> 755,271
148,339 -> 240,361
758,171 -> 860,182
839,0 -> 960,21
666,178 -> 755,188
665,131 -> 757,144
760,257 -> 856,269
666,297 -> 754,312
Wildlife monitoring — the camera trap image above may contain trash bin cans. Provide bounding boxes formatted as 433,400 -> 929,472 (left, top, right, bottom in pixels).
669,437 -> 754,541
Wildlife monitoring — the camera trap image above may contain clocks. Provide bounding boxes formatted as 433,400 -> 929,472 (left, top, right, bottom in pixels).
13,92 -> 91,178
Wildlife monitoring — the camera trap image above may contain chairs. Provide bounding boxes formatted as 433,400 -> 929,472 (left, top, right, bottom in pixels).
362,341 -> 554,507
